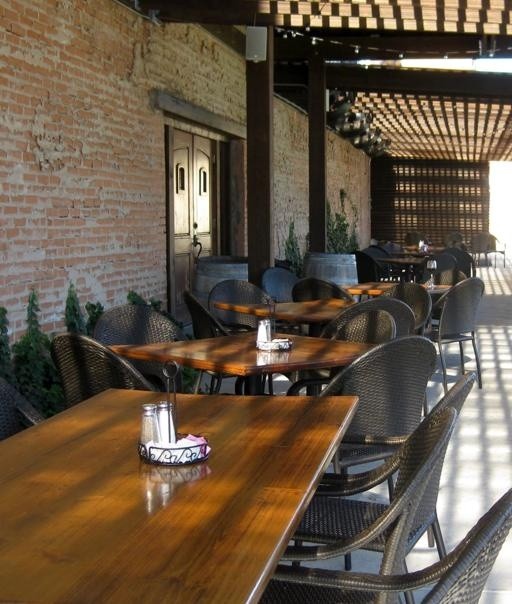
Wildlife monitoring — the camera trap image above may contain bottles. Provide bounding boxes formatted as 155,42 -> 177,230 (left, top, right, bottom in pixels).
157,400 -> 175,444
258,320 -> 271,348
140,404 -> 160,447
